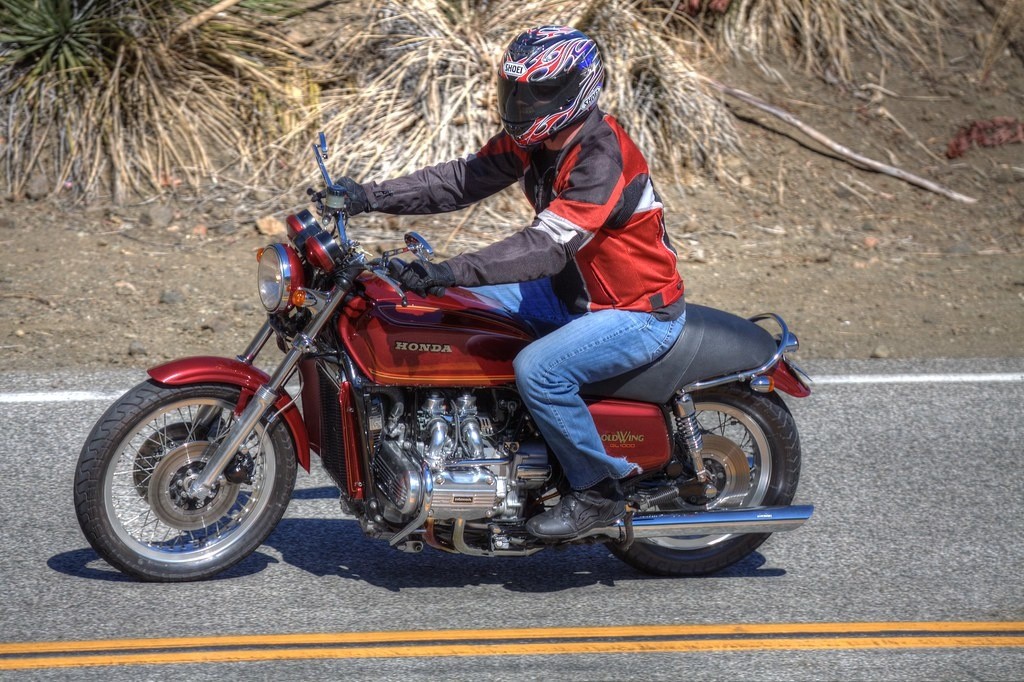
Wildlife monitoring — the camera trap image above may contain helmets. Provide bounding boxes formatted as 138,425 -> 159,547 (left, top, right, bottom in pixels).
498,23 -> 609,148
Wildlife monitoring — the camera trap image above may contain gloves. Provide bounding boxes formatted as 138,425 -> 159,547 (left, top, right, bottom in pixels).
309,178 -> 371,215
398,260 -> 453,296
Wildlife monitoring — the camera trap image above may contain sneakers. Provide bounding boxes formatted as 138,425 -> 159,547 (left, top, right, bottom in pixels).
518,492 -> 628,538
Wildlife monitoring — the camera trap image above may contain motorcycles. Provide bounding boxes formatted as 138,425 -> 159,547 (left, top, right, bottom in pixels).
71,127 -> 816,582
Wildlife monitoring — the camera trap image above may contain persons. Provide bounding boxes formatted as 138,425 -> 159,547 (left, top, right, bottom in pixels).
311,23 -> 688,545
